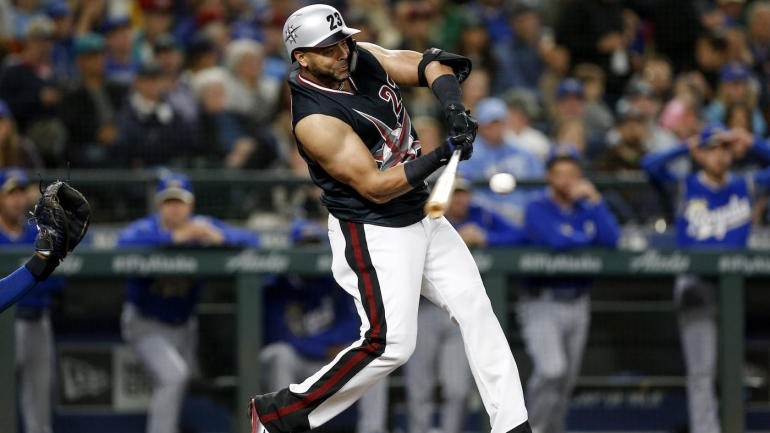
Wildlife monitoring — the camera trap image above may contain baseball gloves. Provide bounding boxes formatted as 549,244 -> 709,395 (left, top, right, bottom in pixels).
31,180 -> 92,257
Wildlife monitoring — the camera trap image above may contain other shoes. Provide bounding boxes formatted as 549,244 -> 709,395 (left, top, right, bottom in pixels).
246,393 -> 287,433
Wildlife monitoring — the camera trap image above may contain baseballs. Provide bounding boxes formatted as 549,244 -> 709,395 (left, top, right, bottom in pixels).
492,171 -> 516,195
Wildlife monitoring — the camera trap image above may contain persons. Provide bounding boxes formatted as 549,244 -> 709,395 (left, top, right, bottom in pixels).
248,3 -> 532,432
0,0 -> 770,433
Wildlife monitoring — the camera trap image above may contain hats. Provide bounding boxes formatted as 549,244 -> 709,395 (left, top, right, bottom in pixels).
478,98 -> 505,124
137,61 -> 169,77
152,171 -> 197,204
44,3 -> 68,18
0,167 -> 29,194
505,87 -> 538,114
545,145 -> 585,167
697,124 -> 730,146
233,26 -> 264,43
453,174 -> 469,189
721,63 -> 747,80
291,221 -> 327,244
101,11 -> 132,35
557,78 -> 588,98
155,35 -> 183,52
71,32 -> 105,54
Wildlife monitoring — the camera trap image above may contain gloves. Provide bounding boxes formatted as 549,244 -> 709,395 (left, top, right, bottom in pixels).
432,103 -> 478,143
435,134 -> 473,166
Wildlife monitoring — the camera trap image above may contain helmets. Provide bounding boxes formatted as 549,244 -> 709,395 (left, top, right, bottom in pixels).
283,4 -> 361,63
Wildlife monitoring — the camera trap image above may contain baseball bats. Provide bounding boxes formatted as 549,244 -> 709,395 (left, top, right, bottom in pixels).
423,148 -> 463,219
